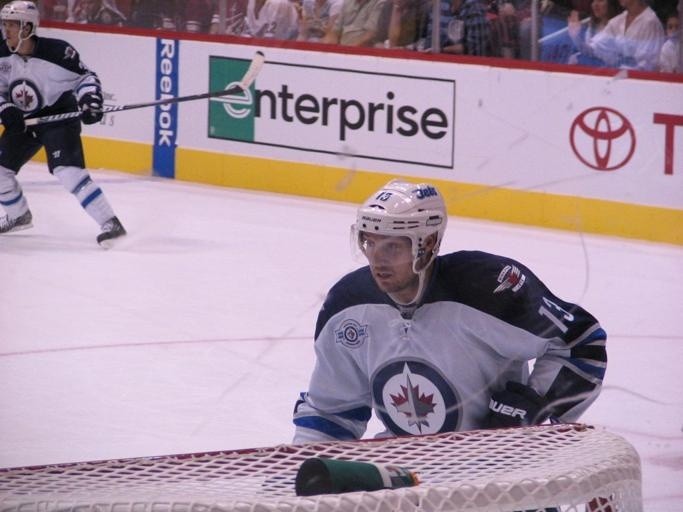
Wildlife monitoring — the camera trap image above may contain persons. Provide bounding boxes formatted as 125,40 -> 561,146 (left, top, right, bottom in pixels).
293,177 -> 608,444
37,1 -> 682,70
0,0 -> 126,243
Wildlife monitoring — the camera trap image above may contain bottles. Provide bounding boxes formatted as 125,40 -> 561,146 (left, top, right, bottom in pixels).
208,15 -> 222,34
295,457 -> 421,497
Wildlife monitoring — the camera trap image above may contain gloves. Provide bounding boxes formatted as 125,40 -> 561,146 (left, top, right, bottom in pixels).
78,98 -> 104,125
489,383 -> 549,430
0,101 -> 26,135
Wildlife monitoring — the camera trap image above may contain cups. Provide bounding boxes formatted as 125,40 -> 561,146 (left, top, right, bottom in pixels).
185,20 -> 201,33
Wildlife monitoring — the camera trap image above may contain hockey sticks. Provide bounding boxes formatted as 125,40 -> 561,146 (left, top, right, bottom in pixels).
0,51 -> 265,136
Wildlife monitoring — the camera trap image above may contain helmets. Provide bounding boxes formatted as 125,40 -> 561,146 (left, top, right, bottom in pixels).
348,177 -> 447,275
1,0 -> 39,42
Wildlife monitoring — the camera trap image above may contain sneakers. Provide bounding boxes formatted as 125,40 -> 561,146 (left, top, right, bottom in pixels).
0,210 -> 31,235
96,216 -> 127,245
